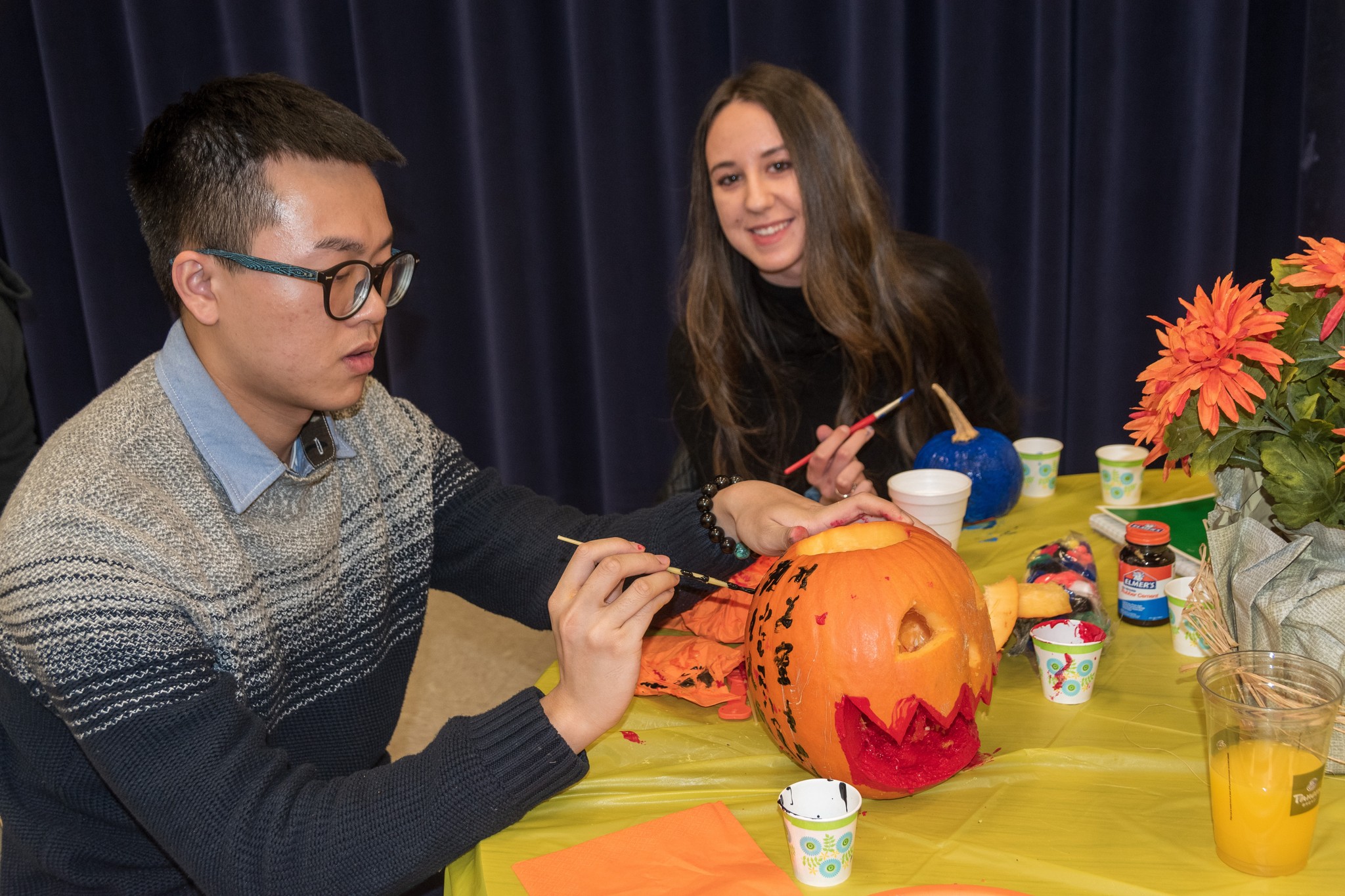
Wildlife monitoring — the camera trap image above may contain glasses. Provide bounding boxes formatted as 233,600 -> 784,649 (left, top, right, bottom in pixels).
198,246 -> 420,322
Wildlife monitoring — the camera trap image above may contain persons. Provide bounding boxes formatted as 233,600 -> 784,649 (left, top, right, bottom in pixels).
2,76 -> 962,896
669,65 -> 1009,507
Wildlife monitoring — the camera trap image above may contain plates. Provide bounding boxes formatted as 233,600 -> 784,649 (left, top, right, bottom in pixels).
867,884 -> 1035,896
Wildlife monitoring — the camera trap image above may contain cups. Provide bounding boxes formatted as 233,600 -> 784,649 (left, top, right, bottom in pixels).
777,778 -> 862,886
887,469 -> 972,553
1030,619 -> 1107,704
1012,437 -> 1064,497
1096,444 -> 1149,505
1196,650 -> 1345,877
1164,576 -> 1217,658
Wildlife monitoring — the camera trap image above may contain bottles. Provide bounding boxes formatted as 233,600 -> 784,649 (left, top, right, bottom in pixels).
1116,520 -> 1176,629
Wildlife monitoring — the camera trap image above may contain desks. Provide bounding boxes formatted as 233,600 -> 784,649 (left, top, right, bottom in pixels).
444,470 -> 1345,896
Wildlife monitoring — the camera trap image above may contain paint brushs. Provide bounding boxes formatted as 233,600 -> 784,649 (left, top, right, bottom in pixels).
784,388 -> 915,474
556,534 -> 756,594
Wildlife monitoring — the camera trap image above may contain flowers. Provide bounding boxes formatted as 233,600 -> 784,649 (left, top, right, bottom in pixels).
1124,235 -> 1345,526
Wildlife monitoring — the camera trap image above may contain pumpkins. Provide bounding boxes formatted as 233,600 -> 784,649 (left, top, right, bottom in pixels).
913,383 -> 1023,524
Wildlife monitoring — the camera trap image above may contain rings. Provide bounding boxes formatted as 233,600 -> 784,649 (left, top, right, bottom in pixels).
834,484 -> 858,499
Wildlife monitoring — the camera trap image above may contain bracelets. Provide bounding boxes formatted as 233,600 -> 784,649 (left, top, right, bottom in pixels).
697,473 -> 758,558
